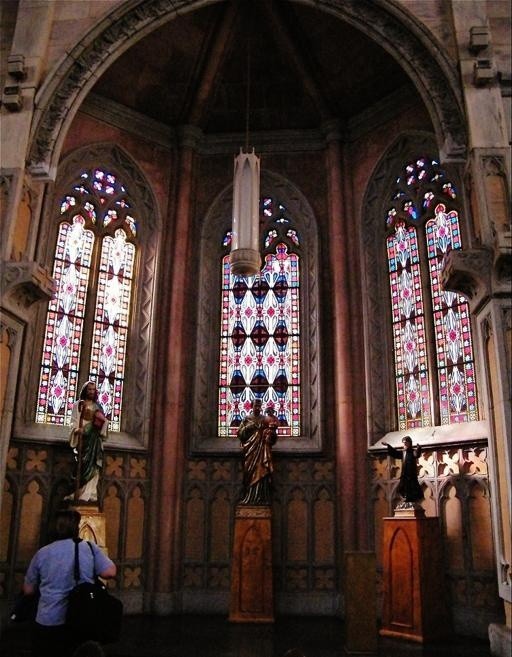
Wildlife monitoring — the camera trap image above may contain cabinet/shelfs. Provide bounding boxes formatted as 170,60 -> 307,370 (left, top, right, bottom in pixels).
225,506 -> 277,624
380,517 -> 441,642
63,505 -> 106,589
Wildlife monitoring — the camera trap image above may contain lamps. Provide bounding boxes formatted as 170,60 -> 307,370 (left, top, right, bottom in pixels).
230,4 -> 261,274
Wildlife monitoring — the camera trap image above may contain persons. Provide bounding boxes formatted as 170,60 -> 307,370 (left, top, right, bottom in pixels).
237,399 -> 281,505
21,508 -> 118,656
381,436 -> 425,509
63,381 -> 107,502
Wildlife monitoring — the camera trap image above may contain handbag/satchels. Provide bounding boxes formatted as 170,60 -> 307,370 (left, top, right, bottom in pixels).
66,582 -> 123,644
